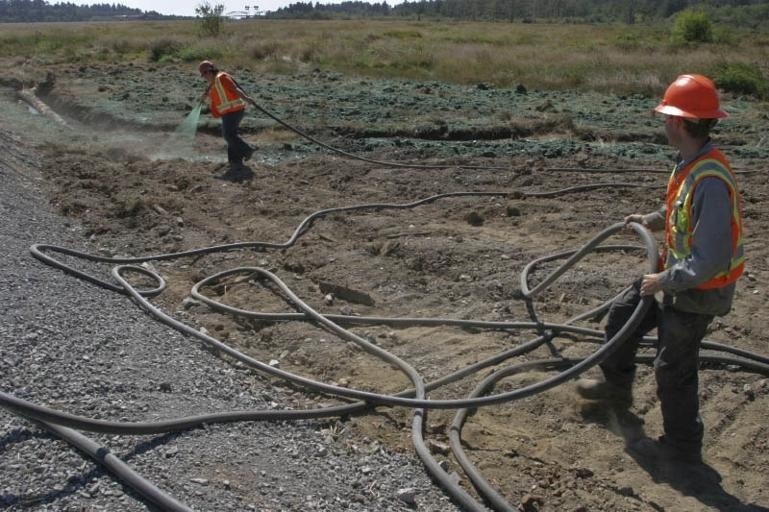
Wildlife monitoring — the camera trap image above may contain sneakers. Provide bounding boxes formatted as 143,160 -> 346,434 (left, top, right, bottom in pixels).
230,147 -> 253,173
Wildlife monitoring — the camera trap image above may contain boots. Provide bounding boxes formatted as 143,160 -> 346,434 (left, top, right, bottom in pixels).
575,363 -> 636,401
629,422 -> 703,473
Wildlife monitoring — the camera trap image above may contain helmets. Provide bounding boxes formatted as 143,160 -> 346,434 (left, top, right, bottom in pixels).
654,73 -> 730,119
199,60 -> 213,77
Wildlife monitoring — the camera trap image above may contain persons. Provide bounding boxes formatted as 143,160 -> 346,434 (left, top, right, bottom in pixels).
576,72 -> 747,470
199,60 -> 255,172
